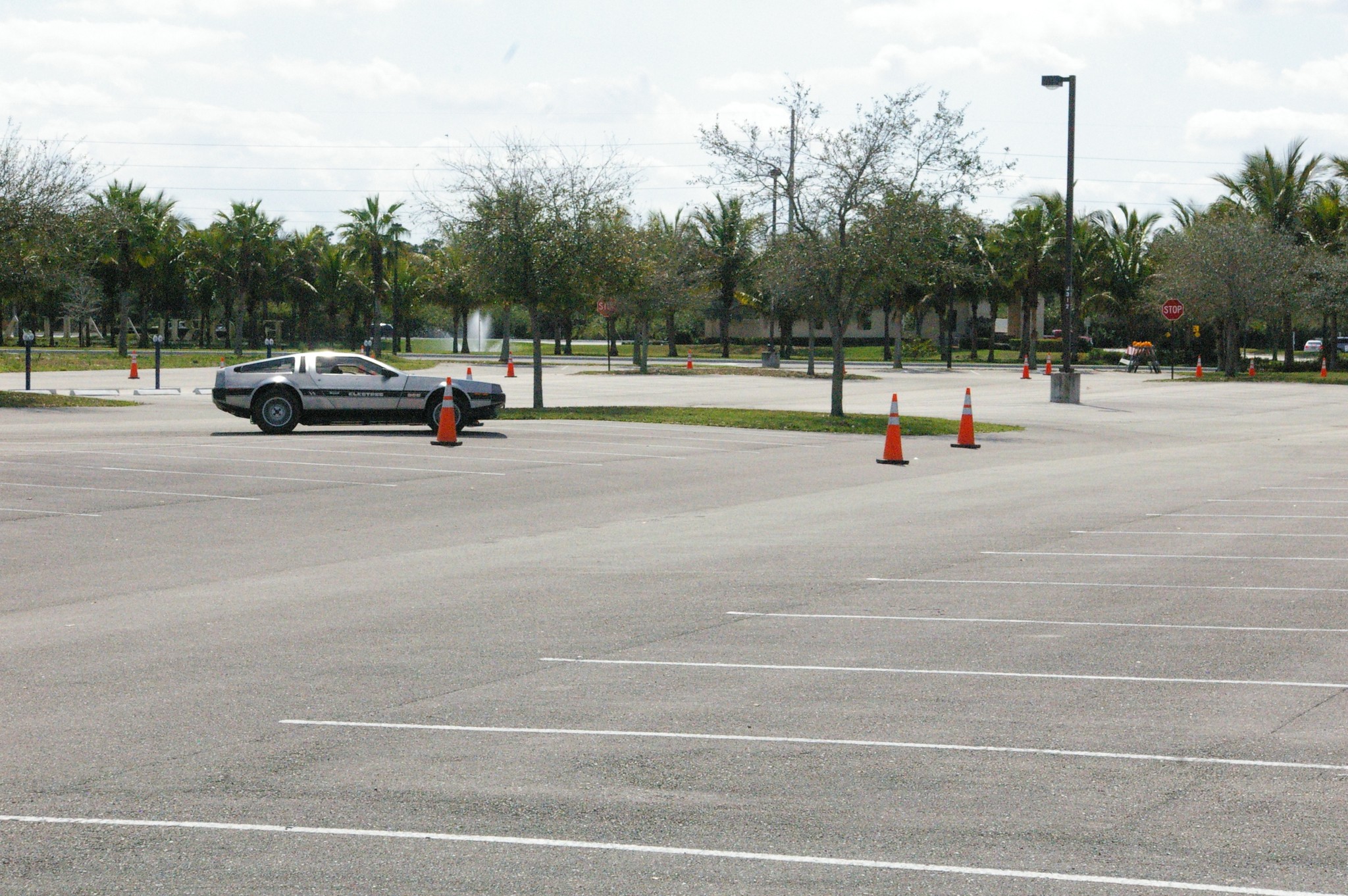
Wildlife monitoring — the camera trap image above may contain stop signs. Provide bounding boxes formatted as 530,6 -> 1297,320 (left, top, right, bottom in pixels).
1162,298 -> 1183,321
596,296 -> 616,317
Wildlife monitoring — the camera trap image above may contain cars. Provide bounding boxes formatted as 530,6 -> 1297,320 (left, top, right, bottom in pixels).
1336,337 -> 1348,354
1303,340 -> 1323,352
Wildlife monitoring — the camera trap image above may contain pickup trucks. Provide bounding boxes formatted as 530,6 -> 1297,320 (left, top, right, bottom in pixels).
1044,329 -> 1093,349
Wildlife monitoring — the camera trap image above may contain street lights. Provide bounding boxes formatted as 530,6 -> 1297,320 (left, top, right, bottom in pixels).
1042,75 -> 1077,373
767,168 -> 782,353
947,234 -> 959,368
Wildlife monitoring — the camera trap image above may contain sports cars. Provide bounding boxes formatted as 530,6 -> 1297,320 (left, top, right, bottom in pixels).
212,351 -> 505,436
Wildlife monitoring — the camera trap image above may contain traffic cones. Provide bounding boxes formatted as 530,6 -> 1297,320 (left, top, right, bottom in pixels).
369,351 -> 376,375
950,387 -> 982,448
220,357 -> 224,369
357,345 -> 366,373
430,377 -> 463,446
1195,356 -> 1202,378
465,367 -> 484,426
687,348 -> 692,368
1249,359 -> 1255,376
1020,355 -> 1032,379
1321,358 -> 1327,377
505,351 -> 517,378
128,351 -> 140,378
1043,352 -> 1052,375
876,393 -> 909,465
